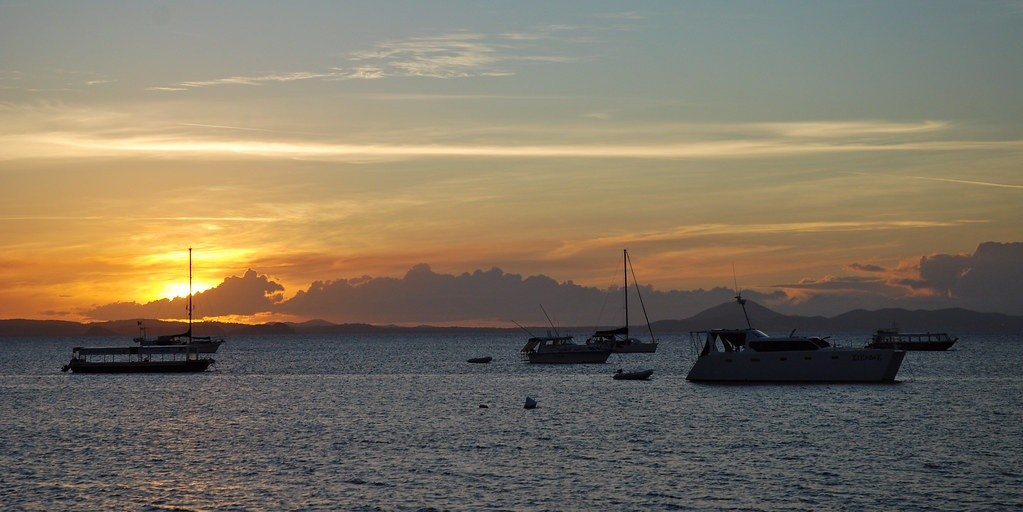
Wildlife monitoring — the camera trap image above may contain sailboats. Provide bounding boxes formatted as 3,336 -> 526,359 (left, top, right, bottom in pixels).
132,246 -> 225,355
594,247 -> 659,355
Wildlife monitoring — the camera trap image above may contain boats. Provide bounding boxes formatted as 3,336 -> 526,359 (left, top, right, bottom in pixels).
685,293 -> 907,384
864,327 -> 959,352
61,319 -> 216,373
512,303 -> 613,364
